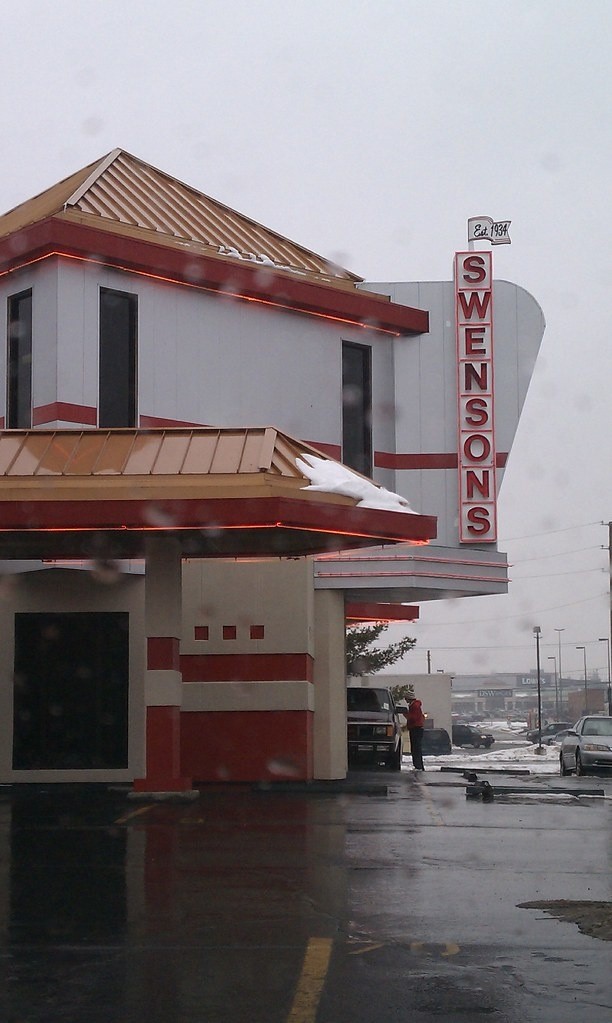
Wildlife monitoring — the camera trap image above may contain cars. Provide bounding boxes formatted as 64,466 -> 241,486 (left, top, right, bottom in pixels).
540,728 -> 572,746
559,715 -> 612,778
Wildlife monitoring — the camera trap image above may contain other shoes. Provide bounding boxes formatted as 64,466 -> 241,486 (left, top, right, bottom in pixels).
411,768 -> 423,771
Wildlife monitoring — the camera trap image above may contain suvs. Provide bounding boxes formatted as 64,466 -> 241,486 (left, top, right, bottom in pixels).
346,685 -> 408,771
420,728 -> 451,756
451,724 -> 496,748
526,722 -> 572,744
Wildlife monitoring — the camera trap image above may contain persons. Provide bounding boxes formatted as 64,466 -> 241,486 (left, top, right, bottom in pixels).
401,689 -> 425,771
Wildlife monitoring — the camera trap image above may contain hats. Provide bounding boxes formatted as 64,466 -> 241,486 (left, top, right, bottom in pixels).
405,689 -> 416,700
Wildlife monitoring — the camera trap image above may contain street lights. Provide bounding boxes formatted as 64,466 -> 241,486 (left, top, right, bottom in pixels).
576,646 -> 587,710
548,656 -> 558,712
599,637 -> 610,688
532,625 -> 546,755
554,628 -> 564,711
